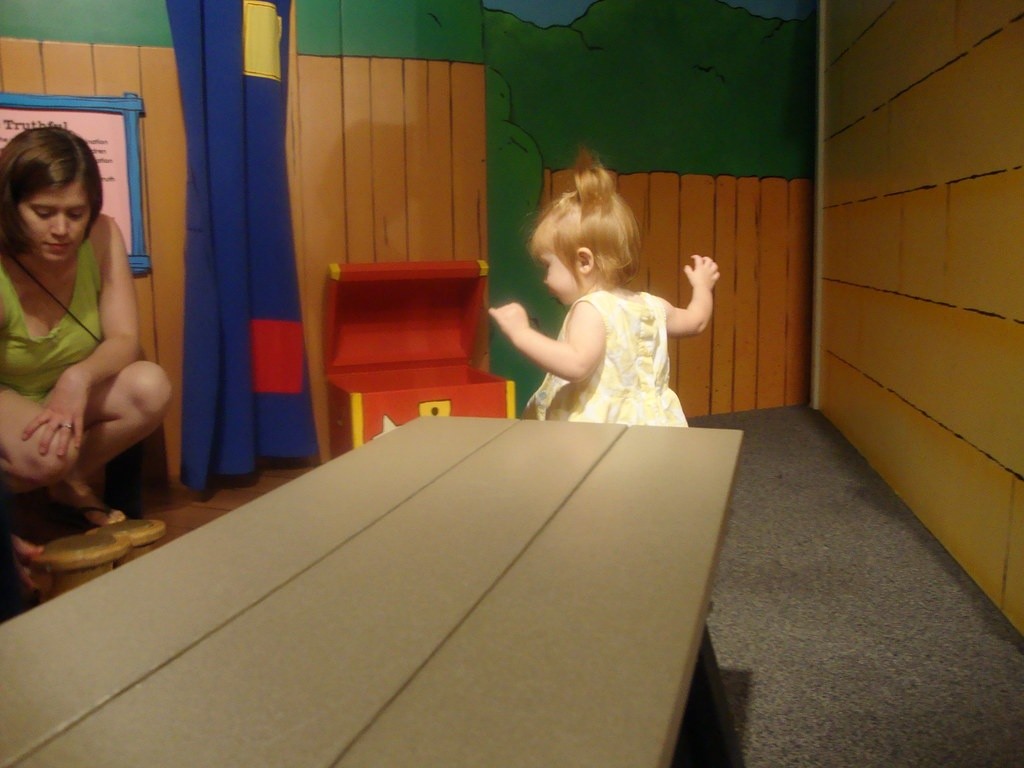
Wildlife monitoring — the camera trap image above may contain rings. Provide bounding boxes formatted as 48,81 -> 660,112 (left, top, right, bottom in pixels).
60,423 -> 73,429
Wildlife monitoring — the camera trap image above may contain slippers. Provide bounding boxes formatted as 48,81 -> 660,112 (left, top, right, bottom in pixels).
40,494 -> 129,528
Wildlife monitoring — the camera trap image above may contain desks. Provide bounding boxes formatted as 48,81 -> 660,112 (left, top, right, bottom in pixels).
0,416 -> 748,768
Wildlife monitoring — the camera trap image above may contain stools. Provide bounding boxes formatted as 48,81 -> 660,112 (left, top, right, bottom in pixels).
28,531 -> 132,601
86,517 -> 167,567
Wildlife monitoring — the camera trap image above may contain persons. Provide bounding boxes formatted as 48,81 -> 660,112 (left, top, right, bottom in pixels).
0,124 -> 174,590
482,149 -> 720,429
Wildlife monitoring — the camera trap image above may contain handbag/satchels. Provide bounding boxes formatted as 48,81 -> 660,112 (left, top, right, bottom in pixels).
100,445 -> 142,521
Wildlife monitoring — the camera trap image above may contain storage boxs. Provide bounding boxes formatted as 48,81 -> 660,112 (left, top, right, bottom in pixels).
321,259 -> 517,460
